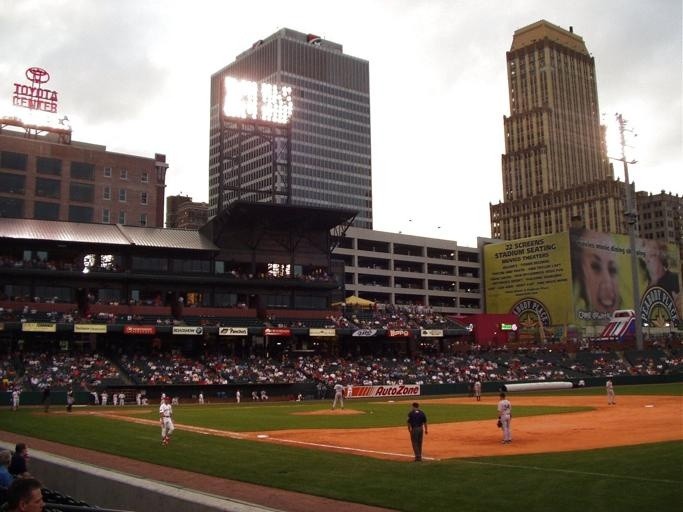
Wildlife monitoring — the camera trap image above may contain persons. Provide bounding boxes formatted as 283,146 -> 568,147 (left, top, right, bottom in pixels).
282,344 -> 507,410
0,450 -> 16,490
159,397 -> 174,448
606,378 -> 616,405
0,478 -> 46,512
480,333 -> 682,381
407,402 -> 427,462
8,444 -> 34,479
643,240 -> 682,312
569,229 -> 626,313
497,392 -> 512,444
2,343 -> 304,413
2,253 -> 446,329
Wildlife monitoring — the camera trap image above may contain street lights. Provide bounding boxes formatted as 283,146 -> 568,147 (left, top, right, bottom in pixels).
607,111 -> 644,351
